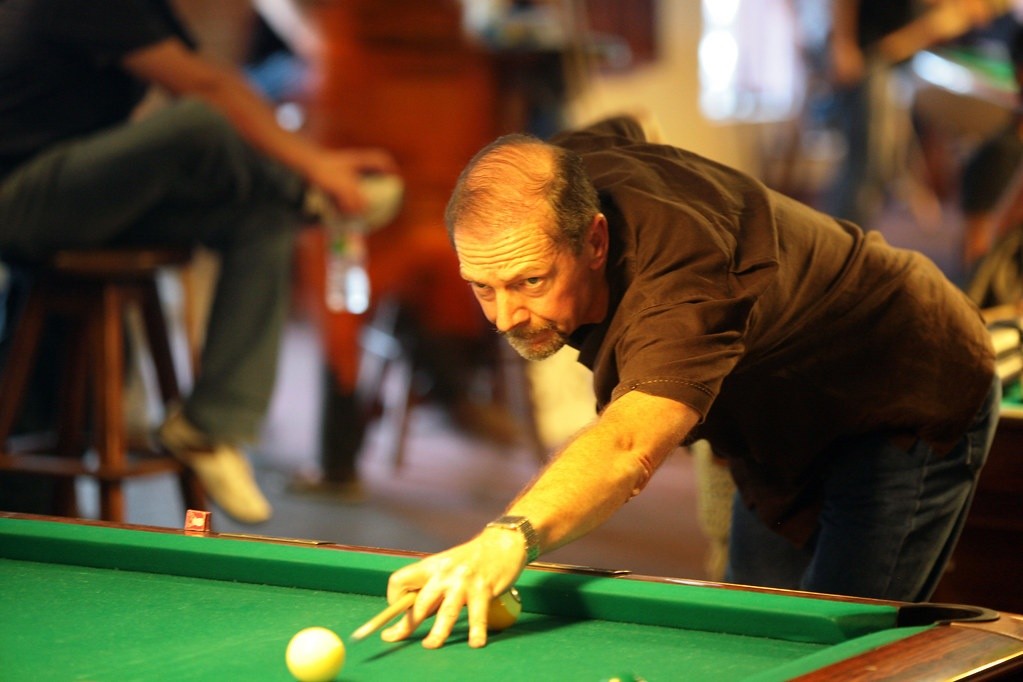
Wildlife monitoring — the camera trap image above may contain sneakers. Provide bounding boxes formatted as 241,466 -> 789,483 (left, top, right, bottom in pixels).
153,408 -> 274,526
301,170 -> 405,238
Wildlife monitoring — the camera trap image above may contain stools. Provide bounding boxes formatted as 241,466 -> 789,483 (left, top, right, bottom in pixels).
0,248 -> 209,531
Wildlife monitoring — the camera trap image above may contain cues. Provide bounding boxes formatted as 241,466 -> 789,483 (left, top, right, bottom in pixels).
352,590 -> 420,641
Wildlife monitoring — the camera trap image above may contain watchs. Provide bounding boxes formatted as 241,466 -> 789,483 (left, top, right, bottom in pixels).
487,515 -> 542,566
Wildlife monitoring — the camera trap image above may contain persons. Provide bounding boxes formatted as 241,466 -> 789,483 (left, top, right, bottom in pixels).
0,0 -> 574,525
821,0 -> 1023,314
383,115 -> 1002,651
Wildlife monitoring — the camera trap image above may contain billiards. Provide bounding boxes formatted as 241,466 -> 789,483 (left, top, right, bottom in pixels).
286,626 -> 346,682
485,587 -> 522,630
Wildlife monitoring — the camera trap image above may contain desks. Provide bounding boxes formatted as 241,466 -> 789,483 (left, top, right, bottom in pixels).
0,510 -> 1023,682
